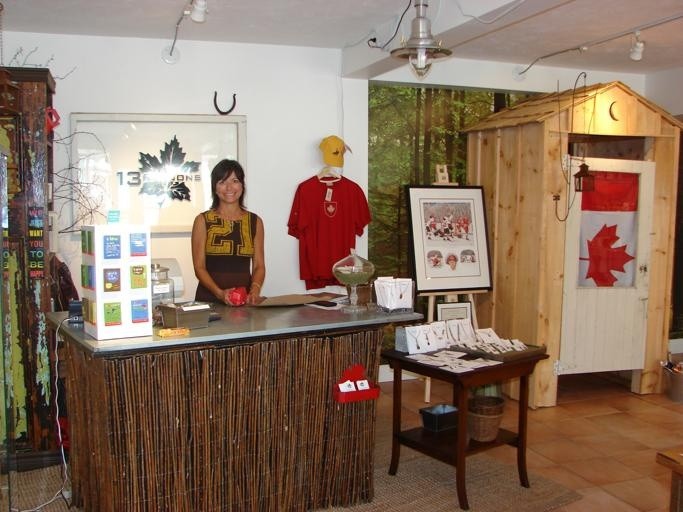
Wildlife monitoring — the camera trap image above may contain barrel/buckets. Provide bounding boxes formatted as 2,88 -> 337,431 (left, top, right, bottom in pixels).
468,396 -> 505,442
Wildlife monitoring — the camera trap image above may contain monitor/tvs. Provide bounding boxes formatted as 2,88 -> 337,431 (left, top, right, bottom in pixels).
50,254 -> 79,311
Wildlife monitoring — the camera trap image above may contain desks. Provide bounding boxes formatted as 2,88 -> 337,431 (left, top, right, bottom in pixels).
384,344 -> 549,508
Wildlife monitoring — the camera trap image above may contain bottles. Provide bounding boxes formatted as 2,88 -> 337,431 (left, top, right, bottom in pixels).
151,261 -> 174,329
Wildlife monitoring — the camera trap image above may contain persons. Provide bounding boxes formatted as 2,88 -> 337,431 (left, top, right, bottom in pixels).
192,159 -> 265,306
426,214 -> 470,241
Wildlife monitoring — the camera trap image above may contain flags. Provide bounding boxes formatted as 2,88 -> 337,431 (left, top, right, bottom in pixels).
580,172 -> 639,287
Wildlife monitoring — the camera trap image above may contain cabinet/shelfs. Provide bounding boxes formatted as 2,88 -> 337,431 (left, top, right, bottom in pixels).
2,69 -> 56,472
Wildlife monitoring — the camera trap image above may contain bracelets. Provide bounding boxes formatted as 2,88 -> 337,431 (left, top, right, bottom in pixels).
251,282 -> 260,288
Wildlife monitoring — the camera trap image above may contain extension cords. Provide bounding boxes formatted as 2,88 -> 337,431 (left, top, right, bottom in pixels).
60,488 -> 73,500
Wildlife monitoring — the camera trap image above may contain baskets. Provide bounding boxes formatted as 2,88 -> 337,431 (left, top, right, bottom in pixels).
467,395 -> 505,442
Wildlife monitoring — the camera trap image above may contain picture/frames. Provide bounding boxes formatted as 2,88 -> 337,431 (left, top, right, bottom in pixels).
73,111 -> 248,236
406,186 -> 493,292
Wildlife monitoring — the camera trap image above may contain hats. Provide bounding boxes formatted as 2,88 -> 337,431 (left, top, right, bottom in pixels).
319,135 -> 345,166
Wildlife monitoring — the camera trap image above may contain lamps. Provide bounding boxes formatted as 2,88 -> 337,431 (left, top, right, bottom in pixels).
160,0 -> 211,65
367,0 -> 453,78
629,32 -> 646,62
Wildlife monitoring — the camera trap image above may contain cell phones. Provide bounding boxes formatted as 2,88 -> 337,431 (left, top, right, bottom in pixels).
316,300 -> 337,307
